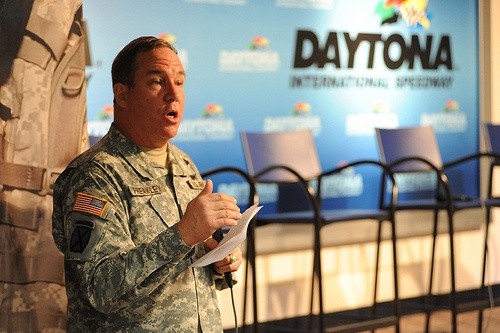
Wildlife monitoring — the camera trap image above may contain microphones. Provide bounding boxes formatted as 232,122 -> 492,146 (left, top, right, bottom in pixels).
438,192 -> 472,202
213,227 -> 233,288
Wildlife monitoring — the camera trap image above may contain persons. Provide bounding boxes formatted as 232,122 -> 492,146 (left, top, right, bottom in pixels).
52,36 -> 243,333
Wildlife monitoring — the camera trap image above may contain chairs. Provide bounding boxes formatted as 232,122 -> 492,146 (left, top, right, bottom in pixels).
243,165 -> 325,333
198,166 -> 259,333
373,156 -> 457,332
310,160 -> 400,333
427,153 -> 500,333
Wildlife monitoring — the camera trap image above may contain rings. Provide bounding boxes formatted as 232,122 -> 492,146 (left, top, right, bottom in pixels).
229,253 -> 237,264
